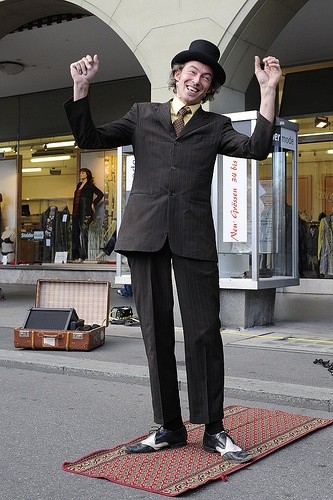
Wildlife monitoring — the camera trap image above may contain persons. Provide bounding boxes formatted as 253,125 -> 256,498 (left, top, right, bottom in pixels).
1,226 -> 15,263
62,40 -> 283,462
96,231 -> 116,259
72,168 -> 105,264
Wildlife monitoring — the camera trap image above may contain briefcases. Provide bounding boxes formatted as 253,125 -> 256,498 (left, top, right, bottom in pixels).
13,278 -> 110,351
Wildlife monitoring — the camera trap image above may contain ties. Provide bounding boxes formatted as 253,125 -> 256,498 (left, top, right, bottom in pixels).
172,106 -> 190,133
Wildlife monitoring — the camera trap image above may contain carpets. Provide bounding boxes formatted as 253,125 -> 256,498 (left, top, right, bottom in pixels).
61,403 -> 333,496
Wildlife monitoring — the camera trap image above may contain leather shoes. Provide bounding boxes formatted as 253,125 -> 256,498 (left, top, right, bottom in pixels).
203,429 -> 252,462
123,425 -> 187,453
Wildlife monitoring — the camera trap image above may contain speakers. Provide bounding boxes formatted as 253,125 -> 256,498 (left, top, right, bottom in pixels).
22,307 -> 79,330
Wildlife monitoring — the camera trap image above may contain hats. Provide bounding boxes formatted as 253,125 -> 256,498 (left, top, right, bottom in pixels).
171,40 -> 227,87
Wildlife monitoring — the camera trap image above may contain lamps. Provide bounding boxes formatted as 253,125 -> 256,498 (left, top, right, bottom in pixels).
0,60 -> 25,75
315,116 -> 331,128
